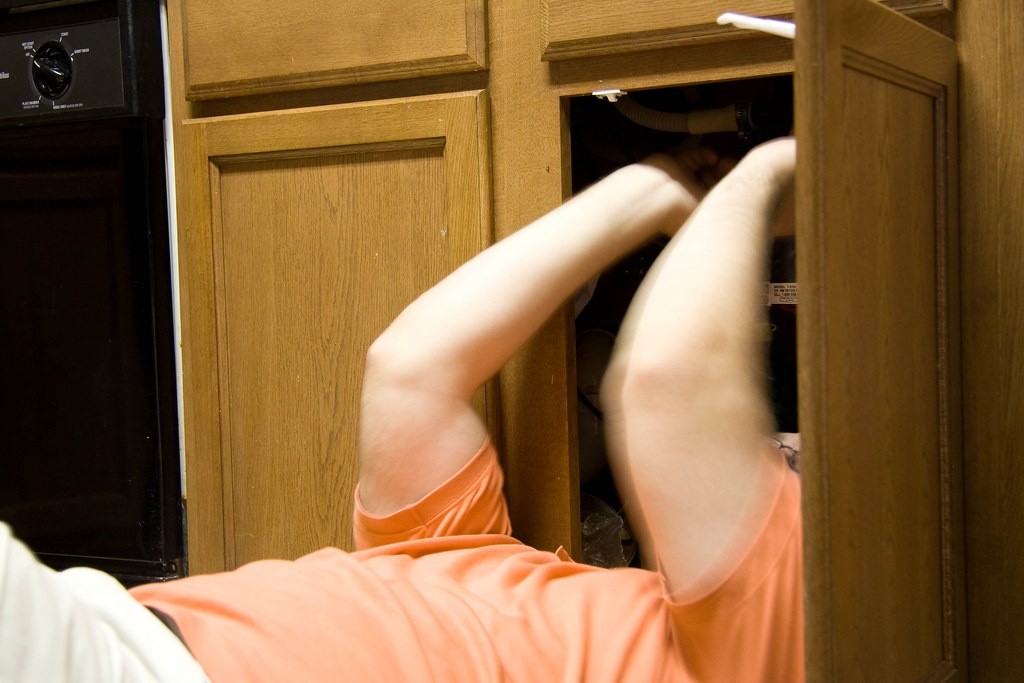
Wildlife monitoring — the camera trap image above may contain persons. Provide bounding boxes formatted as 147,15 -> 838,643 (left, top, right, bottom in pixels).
1,136 -> 805,683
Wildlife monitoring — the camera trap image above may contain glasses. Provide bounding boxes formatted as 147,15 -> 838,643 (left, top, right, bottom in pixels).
771,437 -> 800,473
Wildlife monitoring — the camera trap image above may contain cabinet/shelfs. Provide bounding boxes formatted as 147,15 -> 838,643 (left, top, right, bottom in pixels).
166,0 -> 1024,683
